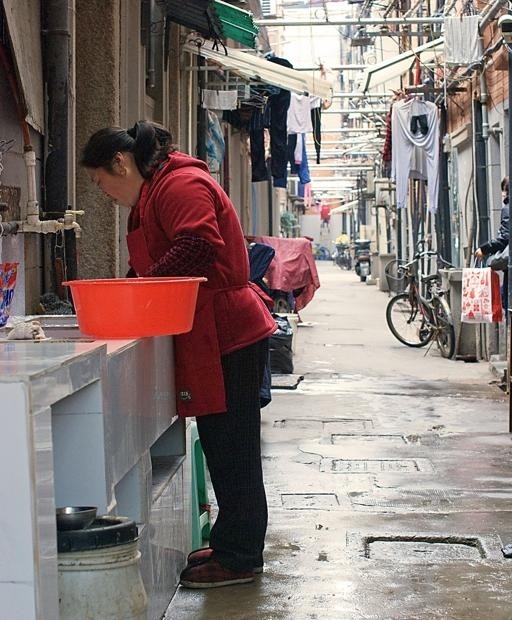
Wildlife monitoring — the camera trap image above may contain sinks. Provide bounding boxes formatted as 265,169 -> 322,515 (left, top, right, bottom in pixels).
28,314 -> 76,327
11,325 -> 89,343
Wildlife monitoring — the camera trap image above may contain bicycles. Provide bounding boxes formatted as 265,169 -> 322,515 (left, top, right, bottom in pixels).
384,240 -> 457,359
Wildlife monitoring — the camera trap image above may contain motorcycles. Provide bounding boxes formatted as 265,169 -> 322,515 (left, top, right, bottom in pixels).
335,241 -> 352,270
267,288 -> 305,314
350,238 -> 375,281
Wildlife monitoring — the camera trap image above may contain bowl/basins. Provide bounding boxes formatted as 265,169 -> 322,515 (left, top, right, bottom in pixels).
61,277 -> 208,340
56,506 -> 97,531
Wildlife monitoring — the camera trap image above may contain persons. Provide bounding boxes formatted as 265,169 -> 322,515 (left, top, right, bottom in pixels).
320,205 -> 331,229
79,116 -> 280,590
474,175 -> 510,326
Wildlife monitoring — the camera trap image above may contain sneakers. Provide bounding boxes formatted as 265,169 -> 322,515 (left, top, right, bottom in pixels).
180,546 -> 264,588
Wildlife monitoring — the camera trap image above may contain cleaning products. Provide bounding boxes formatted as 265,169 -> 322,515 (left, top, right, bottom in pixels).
2,259 -> 20,324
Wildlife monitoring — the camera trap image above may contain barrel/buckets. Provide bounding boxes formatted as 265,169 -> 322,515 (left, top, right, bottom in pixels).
57,516 -> 151,616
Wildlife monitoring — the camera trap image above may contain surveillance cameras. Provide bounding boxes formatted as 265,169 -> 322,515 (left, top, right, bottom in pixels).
497,15 -> 512,36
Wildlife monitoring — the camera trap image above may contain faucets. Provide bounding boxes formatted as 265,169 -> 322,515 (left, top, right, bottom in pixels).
56,206 -> 85,238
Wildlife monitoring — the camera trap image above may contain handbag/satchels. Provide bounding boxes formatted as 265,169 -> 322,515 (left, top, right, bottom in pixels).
461,257 -> 502,324
